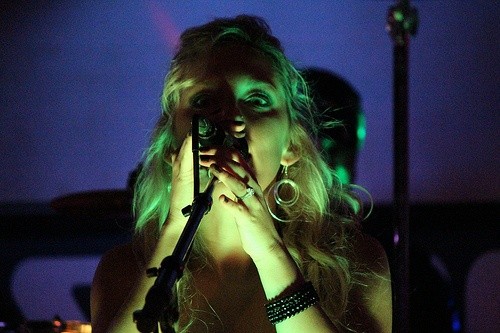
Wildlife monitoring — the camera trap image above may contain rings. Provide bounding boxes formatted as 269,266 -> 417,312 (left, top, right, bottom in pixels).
239,188 -> 254,201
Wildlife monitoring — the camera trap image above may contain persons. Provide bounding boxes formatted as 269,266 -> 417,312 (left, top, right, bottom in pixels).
89,14 -> 393,332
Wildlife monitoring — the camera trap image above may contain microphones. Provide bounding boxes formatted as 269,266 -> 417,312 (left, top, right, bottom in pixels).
193,115 -> 248,158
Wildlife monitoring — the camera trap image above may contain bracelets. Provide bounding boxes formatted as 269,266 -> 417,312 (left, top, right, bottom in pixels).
263,280 -> 320,328
